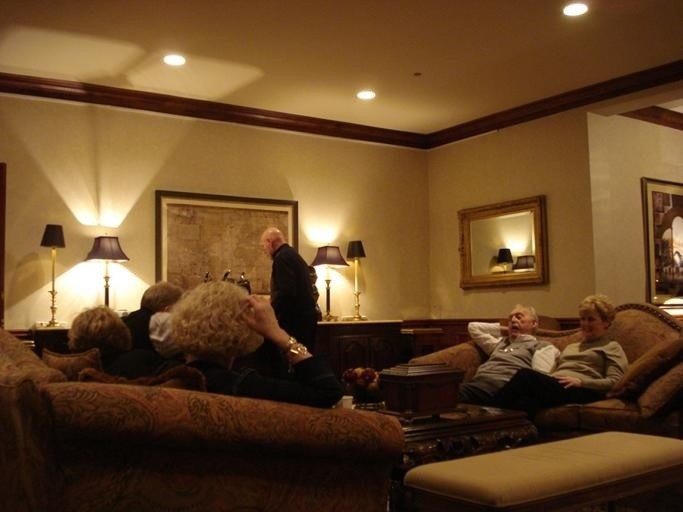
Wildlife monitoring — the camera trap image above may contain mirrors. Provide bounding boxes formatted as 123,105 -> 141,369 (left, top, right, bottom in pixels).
456,194 -> 549,288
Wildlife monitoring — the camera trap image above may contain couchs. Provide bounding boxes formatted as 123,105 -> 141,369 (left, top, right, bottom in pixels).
406,301 -> 682,440
0,329 -> 404,509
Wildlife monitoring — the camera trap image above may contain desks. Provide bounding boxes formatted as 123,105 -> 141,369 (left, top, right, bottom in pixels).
378,362 -> 465,411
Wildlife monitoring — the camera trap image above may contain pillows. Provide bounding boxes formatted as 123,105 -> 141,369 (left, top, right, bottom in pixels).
77,366 -> 207,394
39,345 -> 103,382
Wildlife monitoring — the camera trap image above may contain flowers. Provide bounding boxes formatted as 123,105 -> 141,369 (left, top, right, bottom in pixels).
342,365 -> 382,393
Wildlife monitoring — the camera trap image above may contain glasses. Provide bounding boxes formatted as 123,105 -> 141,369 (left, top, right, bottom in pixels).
499,347 -> 518,353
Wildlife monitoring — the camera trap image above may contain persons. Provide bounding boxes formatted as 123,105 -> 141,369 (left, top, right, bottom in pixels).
466,293 -> 629,451
260,226 -> 317,356
50,282 -> 344,409
457,304 -> 561,407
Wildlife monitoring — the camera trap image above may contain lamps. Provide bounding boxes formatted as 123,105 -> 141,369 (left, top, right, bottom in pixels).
305,246 -> 350,320
82,234 -> 129,309
489,245 -> 535,274
39,223 -> 65,327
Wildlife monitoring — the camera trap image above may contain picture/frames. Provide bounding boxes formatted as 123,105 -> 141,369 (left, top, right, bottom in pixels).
640,176 -> 682,308
154,188 -> 298,303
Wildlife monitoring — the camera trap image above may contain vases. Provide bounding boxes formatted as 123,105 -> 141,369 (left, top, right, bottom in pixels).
351,387 -> 385,403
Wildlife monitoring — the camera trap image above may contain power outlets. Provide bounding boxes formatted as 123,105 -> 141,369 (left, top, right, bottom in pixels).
343,240 -> 366,322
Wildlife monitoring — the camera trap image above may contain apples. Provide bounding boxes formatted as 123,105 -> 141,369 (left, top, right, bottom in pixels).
341,367 -> 381,392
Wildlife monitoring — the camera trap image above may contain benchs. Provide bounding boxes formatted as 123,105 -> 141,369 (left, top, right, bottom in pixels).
404,430 -> 682,510
